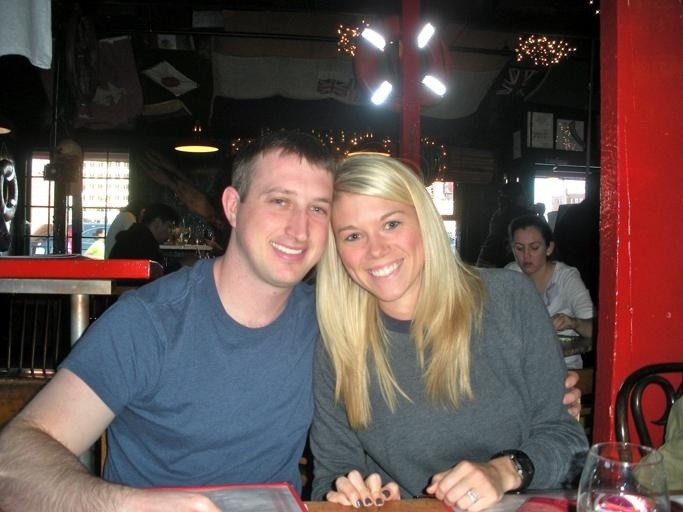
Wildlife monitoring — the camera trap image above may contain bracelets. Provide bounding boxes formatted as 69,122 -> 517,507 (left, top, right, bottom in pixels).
510,454 -> 523,480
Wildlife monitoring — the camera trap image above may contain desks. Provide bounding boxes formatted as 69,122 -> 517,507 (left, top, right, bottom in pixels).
159,242 -> 213,251
302,492 -> 529,512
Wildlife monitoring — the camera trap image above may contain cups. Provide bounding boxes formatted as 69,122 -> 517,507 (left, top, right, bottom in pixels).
574,440 -> 671,512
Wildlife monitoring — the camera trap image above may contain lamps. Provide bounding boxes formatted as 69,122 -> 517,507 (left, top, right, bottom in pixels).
514,33 -> 576,71
174,125 -> 220,153
336,20 -> 455,109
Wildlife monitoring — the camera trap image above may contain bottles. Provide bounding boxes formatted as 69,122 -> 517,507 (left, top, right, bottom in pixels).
166,216 -> 214,245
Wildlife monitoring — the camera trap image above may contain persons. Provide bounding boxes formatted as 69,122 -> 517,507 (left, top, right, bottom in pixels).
476,182 -> 552,268
554,172 -> 600,367
503,216 -> 597,370
108,204 -> 198,295
105,201 -> 152,259
309,154 -> 589,512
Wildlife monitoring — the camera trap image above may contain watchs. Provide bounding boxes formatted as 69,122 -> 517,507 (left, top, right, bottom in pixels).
0,133 -> 582,512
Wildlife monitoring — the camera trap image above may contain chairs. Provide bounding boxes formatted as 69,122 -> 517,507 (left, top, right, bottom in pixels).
615,362 -> 683,485
